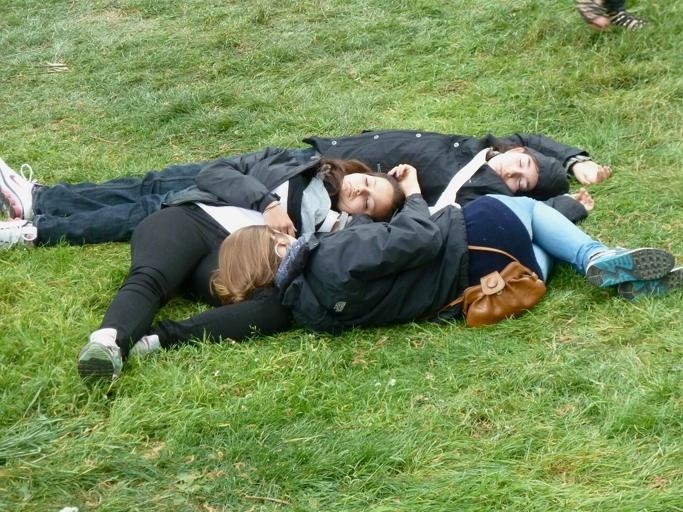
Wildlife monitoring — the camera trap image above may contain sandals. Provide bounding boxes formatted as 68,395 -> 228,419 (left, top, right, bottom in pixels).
575,0 -> 646,31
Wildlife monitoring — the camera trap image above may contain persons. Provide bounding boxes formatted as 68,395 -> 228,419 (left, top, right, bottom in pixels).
574,0 -> 649,31
214,164 -> 682,330
0,130 -> 613,247
78,159 -> 431,397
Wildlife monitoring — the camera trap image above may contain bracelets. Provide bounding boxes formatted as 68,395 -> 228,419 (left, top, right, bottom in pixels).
264,203 -> 281,211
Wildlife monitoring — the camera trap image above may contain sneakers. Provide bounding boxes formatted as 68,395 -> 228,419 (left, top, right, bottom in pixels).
0,157 -> 38,246
586,248 -> 683,298
130,334 -> 161,359
78,327 -> 123,396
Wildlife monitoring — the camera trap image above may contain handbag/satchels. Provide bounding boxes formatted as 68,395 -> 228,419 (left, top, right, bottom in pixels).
438,245 -> 546,328
